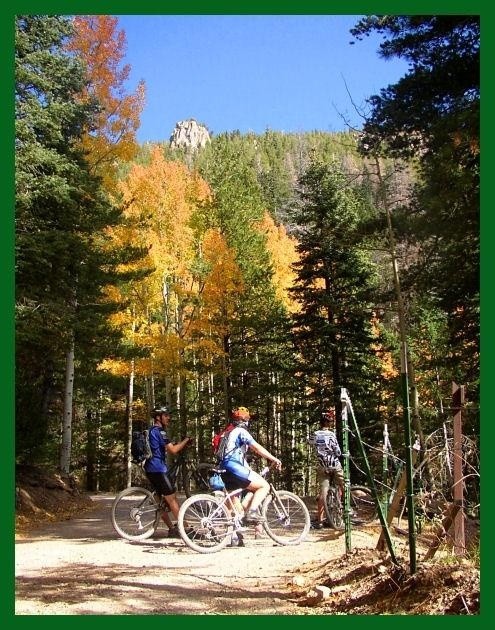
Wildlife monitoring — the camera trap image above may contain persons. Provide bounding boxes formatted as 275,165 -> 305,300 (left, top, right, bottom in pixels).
214,406 -> 283,548
308,417 -> 361,532
143,406 -> 194,538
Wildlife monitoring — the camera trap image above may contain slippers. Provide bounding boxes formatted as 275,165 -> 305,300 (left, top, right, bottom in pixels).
131,427 -> 161,461
213,423 -> 243,460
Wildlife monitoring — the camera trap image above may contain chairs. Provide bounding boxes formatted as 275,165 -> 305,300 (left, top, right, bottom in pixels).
183,527 -> 204,539
244,510 -> 266,522
314,519 -> 324,527
167,527 -> 179,537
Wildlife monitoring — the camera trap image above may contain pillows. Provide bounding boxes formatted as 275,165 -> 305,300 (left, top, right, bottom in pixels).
321,412 -> 334,420
233,407 -> 250,420
149,406 -> 170,417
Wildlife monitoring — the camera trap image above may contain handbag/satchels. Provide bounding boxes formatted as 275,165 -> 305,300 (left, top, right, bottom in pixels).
187,436 -> 191,441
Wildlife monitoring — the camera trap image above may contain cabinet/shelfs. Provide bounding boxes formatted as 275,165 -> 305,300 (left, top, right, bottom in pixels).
233,491 -> 254,516
